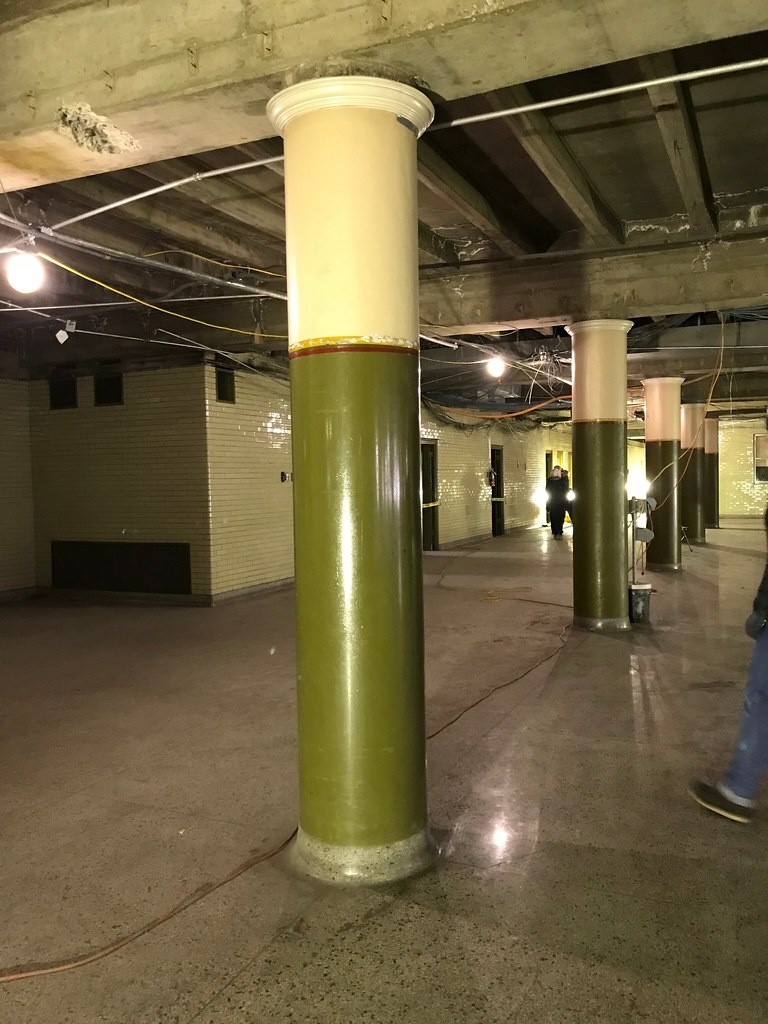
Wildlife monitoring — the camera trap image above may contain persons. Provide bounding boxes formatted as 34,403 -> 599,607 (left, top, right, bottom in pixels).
688,416 -> 768,823
545,466 -> 568,540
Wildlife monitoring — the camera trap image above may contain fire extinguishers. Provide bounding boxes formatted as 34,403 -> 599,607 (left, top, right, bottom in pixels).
488,468 -> 497,487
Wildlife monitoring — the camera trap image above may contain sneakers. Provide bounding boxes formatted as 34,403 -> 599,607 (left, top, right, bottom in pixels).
684,778 -> 760,826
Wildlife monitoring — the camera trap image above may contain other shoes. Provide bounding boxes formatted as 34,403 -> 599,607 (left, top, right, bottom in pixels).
553,533 -> 563,541
